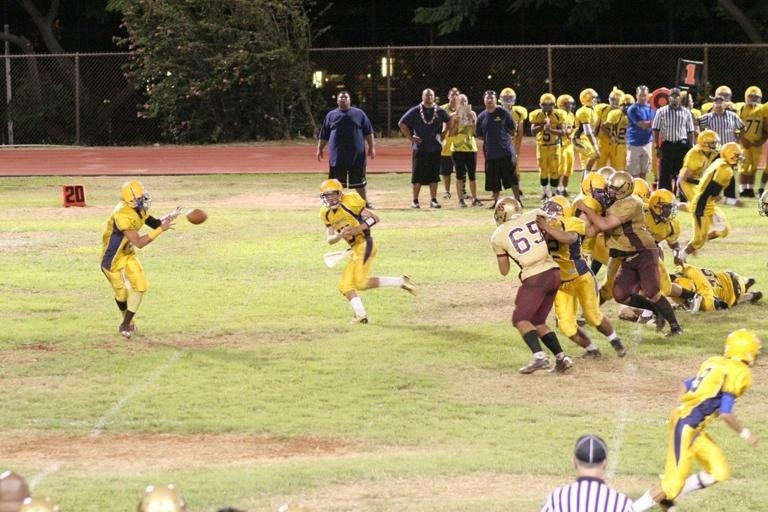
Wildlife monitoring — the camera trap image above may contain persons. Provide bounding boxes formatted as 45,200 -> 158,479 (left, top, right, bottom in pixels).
540,435 -> 634,512
632,329 -> 762,512
100,181 -> 184,339
0,472 -> 28,512
317,91 -> 376,208
319,179 -> 417,325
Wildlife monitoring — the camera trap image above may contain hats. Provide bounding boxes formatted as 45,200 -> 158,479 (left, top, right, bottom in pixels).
668,88 -> 680,97
574,434 -> 608,467
713,95 -> 725,103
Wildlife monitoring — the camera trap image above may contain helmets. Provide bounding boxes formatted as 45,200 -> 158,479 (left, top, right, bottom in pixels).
581,165 -> 635,206
758,189 -> 768,217
633,177 -> 652,202
744,85 -> 762,104
619,94 -> 636,116
697,129 -> 720,154
720,142 -> 744,166
724,327 -> 762,367
671,278 -> 695,307
319,179 -> 344,210
570,196 -> 603,229
579,88 -> 599,108
499,87 -> 516,106
540,195 -> 572,220
120,179 -> 152,211
556,94 -> 575,111
649,188 -> 677,224
680,90 -> 694,103
715,85 -> 733,102
608,89 -> 624,108
539,93 -> 556,113
493,196 -> 523,227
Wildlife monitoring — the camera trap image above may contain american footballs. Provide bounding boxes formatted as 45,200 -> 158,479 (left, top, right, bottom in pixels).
186,209 -> 208,224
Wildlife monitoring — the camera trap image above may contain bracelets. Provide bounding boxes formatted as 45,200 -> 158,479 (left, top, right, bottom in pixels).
739,427 -> 750,440
148,227 -> 162,239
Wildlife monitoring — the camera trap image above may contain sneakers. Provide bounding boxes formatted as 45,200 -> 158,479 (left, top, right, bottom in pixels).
517,356 -> 553,375
750,291 -> 763,306
347,315 -> 369,325
659,497 -> 679,512
519,190 -> 523,195
576,314 -> 586,328
399,273 -> 418,297
654,316 -> 666,333
759,188 -> 764,196
576,349 -> 602,360
430,198 -> 442,209
366,202 -> 372,209
724,196 -> 738,207
542,189 -> 570,200
443,191 -> 451,199
544,356 -> 574,375
664,327 -> 683,338
637,308 -> 654,325
610,337 -> 627,358
411,199 -> 420,208
118,321 -> 137,339
744,278 -> 755,293
458,192 -> 486,208
740,189 -> 756,198
686,293 -> 704,314
